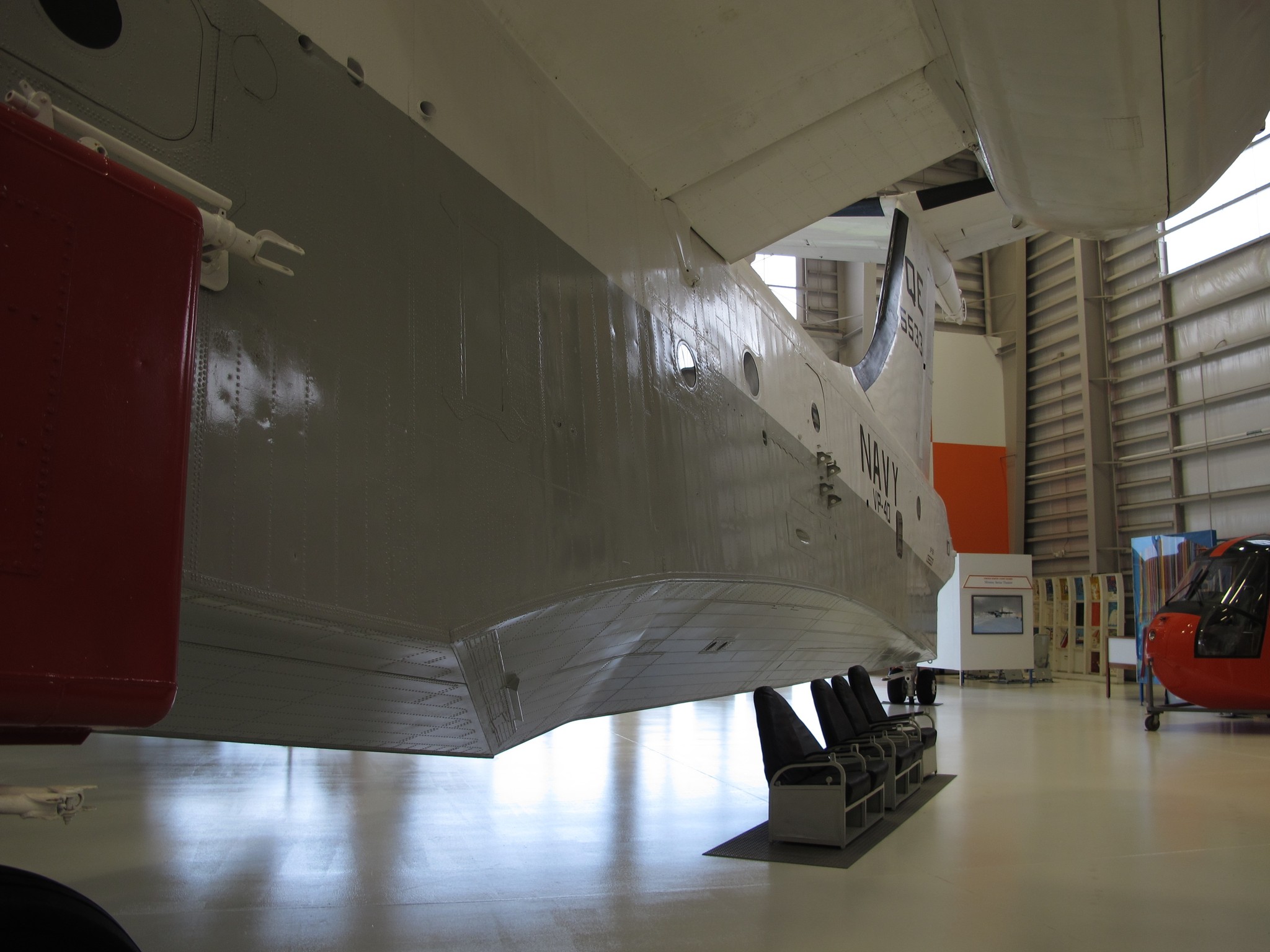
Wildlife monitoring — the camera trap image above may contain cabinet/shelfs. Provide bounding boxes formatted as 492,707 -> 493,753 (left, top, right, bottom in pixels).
1033,572 -> 1125,684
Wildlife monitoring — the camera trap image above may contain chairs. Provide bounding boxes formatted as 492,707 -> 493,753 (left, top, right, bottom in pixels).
753,665 -> 938,849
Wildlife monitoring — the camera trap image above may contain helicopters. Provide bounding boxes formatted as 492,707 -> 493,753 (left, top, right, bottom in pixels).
1140,530 -> 1270,733
